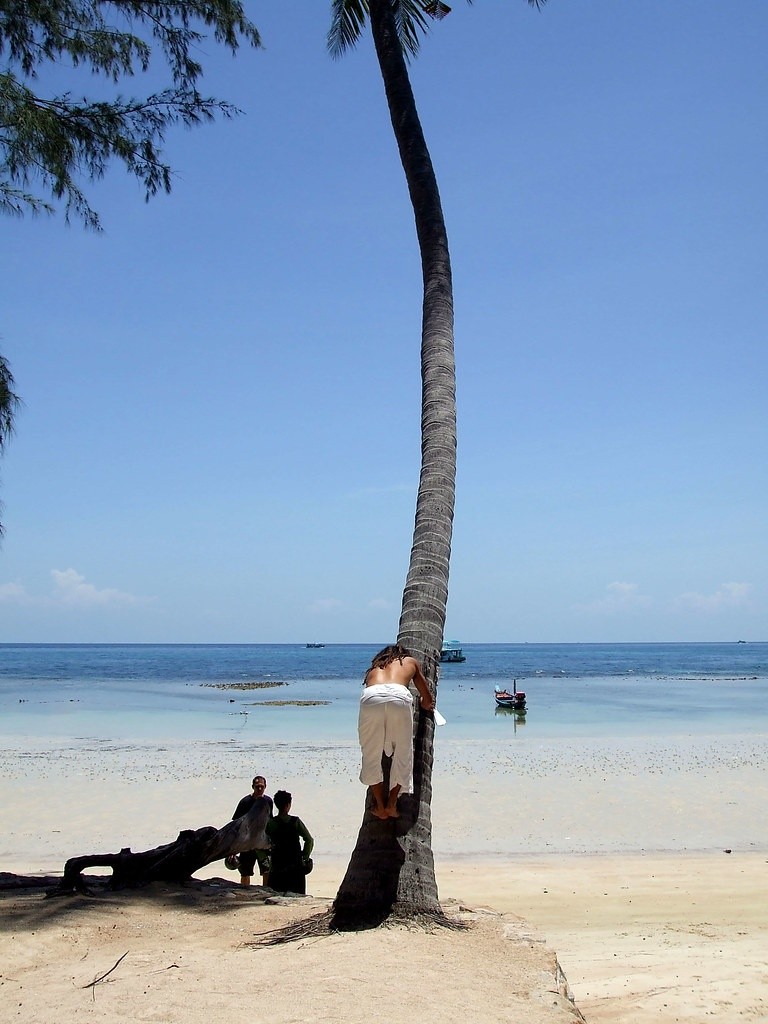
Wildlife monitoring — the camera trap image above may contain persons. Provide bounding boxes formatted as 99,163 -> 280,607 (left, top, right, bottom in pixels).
358,643 -> 433,819
225,776 -> 314,895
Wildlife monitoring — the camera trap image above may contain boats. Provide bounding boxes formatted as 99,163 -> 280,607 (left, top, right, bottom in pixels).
494,678 -> 527,711
438,640 -> 467,661
